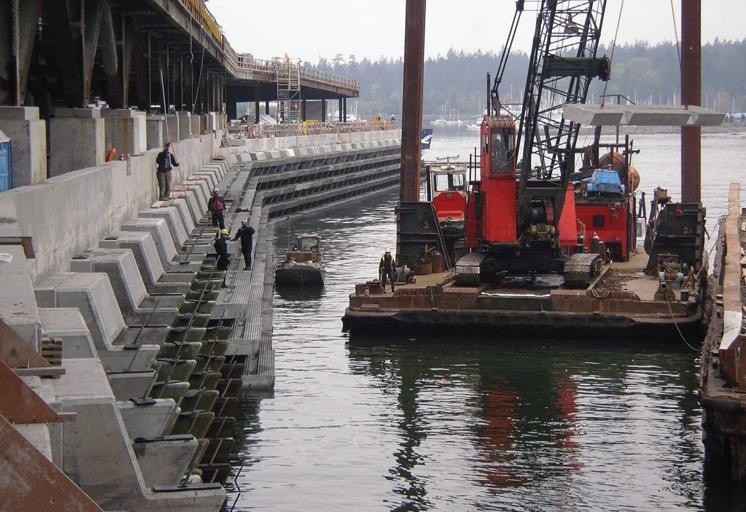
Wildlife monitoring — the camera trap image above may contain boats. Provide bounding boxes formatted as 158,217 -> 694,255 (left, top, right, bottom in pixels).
341,0 -> 711,335
270,215 -> 327,288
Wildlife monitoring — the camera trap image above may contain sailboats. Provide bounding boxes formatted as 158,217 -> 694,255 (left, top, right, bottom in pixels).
325,79 -> 573,134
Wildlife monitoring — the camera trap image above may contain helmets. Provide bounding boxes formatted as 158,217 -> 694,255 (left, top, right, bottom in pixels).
220,229 -> 229,235
385,248 -> 390,253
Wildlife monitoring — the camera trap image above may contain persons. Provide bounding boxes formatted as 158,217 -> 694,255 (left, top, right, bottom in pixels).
207,189 -> 226,230
379,248 -> 395,293
230,219 -> 256,270
214,229 -> 229,287
376,113 -> 384,131
390,115 -> 396,128
155,141 -> 179,201
